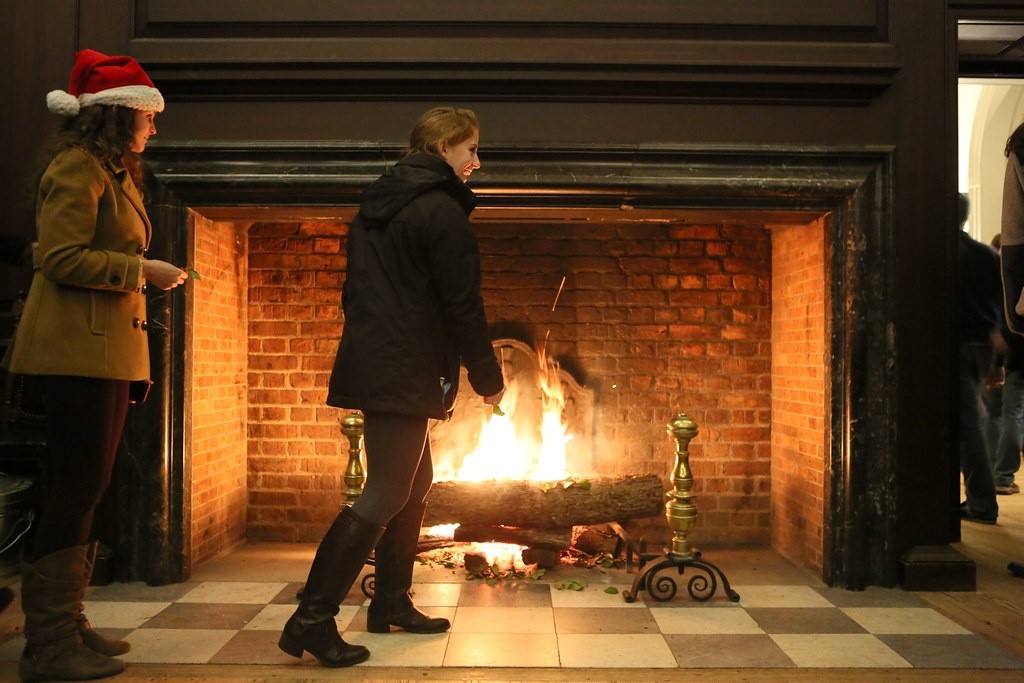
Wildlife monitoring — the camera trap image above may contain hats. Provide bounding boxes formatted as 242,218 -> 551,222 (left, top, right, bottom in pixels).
45,49 -> 165,118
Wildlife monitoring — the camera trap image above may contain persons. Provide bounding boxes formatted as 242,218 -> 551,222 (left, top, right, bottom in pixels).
957,191 -> 1007,526
987,232 -> 1024,494
278,105 -> 507,670
9,48 -> 190,683
1000,122 -> 1024,336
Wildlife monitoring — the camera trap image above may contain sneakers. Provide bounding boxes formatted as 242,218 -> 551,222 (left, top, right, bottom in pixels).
995,481 -> 1019,495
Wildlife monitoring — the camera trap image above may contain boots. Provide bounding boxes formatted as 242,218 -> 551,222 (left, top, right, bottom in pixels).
367,501 -> 451,634
20,542 -> 126,683
81,538 -> 131,658
278,503 -> 388,669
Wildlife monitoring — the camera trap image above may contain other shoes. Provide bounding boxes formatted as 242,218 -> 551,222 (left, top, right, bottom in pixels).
961,510 -> 997,524
1008,561 -> 1024,578
